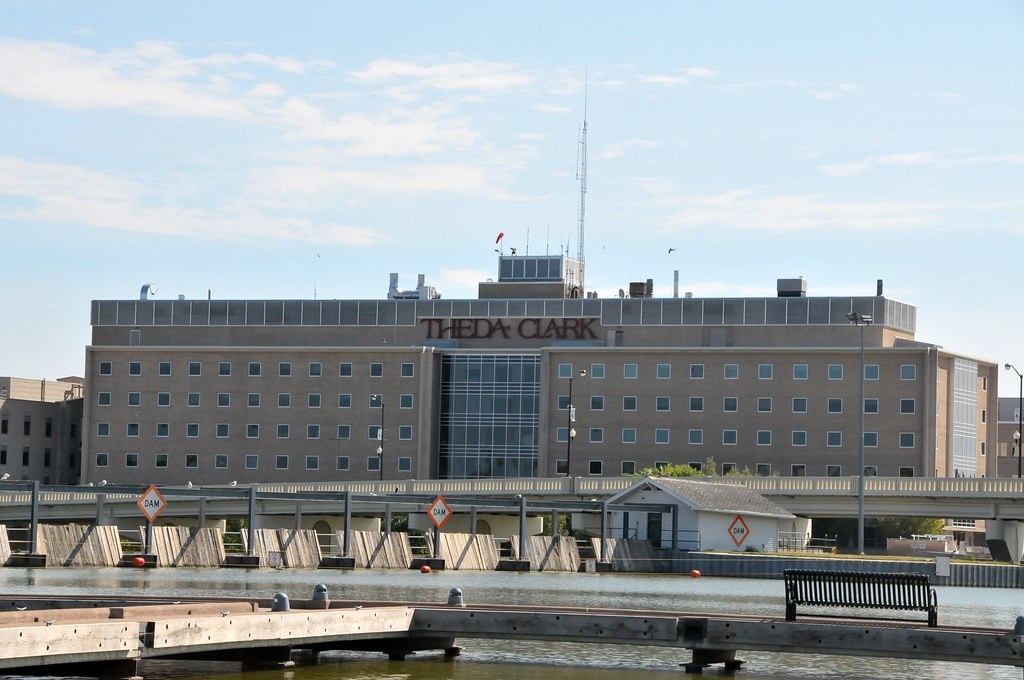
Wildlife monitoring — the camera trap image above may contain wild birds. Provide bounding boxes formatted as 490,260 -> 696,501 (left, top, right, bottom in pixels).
96,479 -> 107,487
228,480 -> 238,488
87,483 -> 93,486
187,480 -> 193,489
0,473 -> 10,481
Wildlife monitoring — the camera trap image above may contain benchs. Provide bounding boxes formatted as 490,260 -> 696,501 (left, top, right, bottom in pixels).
782,568 -> 938,628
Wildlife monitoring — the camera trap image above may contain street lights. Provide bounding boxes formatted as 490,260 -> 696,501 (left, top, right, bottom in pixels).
847,309 -> 873,555
566,370 -> 587,475
1005,362 -> 1023,479
369,393 -> 386,481
